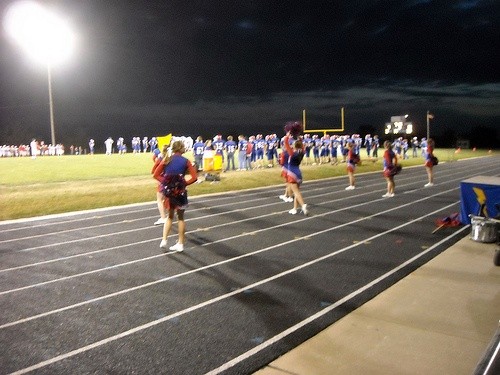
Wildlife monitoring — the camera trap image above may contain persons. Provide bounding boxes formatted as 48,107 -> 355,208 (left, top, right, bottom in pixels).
278,141 -> 294,202
154,141 -> 198,253
424,138 -> 435,187
152,145 -> 170,225
382,141 -> 397,198
0,134 -> 427,173
346,142 -> 357,190
283,129 -> 309,217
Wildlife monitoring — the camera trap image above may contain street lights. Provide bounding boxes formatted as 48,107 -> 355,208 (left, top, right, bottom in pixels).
39,44 -> 58,154
425,111 -> 434,144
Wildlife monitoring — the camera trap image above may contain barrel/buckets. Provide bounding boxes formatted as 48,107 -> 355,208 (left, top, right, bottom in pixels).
471,217 -> 499,242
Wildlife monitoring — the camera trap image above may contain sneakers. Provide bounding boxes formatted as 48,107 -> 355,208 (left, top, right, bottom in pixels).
300,204 -> 308,216
169,242 -> 183,252
154,218 -> 165,224
345,186 -> 355,190
284,198 -> 293,203
160,240 -> 169,250
382,193 -> 391,198
390,193 -> 395,197
424,183 -> 433,187
278,195 -> 288,199
289,209 -> 297,215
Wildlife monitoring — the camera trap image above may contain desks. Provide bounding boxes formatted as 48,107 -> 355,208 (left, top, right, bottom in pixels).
458,175 -> 500,224
